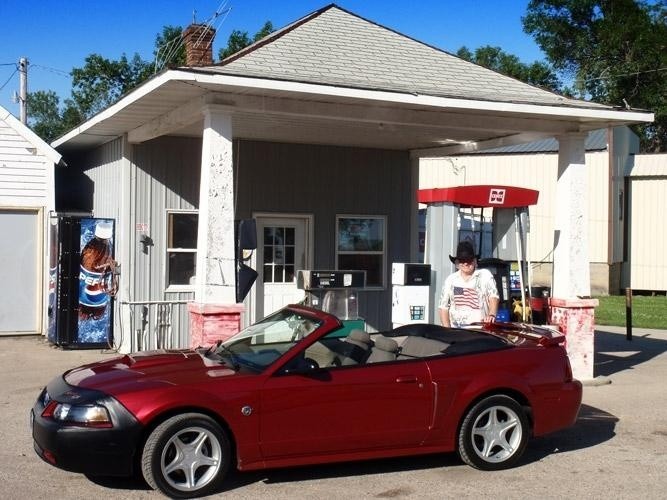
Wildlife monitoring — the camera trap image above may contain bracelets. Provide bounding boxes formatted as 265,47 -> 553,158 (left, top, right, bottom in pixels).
490,314 -> 497,320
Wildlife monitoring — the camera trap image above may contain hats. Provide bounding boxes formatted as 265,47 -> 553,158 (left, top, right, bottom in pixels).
448,241 -> 481,262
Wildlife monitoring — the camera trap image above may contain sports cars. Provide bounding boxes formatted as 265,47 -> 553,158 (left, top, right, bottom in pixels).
30,304 -> 583,500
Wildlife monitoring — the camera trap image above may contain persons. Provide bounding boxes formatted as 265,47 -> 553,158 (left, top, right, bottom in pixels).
513,278 -> 520,289
438,241 -> 500,329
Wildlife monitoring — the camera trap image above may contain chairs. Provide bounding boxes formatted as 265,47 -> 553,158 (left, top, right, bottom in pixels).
364,337 -> 398,362
325,328 -> 370,366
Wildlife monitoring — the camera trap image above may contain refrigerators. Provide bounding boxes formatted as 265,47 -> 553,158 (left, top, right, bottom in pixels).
48,217 -> 116,350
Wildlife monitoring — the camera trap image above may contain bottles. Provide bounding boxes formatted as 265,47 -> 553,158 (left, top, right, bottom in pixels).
77,222 -> 113,321
48,217 -> 58,342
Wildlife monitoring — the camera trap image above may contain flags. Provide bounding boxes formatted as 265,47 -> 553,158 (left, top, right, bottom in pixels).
453,286 -> 480,309
